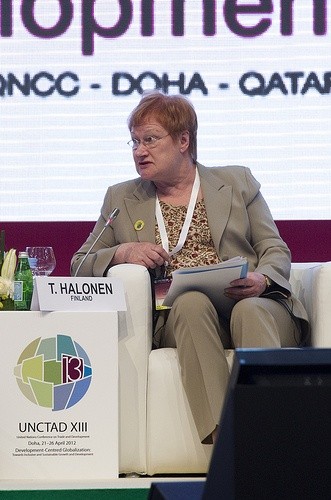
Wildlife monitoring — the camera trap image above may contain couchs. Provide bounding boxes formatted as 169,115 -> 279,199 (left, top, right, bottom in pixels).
110,261 -> 331,476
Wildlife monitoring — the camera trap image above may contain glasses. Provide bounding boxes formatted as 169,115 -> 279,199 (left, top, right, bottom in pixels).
126,131 -> 173,151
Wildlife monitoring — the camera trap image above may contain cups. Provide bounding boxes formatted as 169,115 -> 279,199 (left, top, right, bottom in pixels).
24,246 -> 57,277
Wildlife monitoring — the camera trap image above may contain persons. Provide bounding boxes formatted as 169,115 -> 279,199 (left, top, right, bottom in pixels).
70,93 -> 310,444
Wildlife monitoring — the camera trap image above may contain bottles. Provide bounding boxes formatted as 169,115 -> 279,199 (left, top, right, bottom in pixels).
13,251 -> 40,310
0,229 -> 10,310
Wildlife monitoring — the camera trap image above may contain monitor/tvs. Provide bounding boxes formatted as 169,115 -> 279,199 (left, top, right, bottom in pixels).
196,346 -> 330,500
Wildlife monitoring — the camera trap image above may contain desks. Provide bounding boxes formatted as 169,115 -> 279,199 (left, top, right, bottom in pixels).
1,309 -> 121,480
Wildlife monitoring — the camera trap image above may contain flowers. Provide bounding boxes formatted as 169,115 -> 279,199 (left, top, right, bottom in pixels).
0,248 -> 18,310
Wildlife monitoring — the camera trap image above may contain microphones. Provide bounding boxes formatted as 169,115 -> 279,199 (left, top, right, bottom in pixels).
74,208 -> 119,277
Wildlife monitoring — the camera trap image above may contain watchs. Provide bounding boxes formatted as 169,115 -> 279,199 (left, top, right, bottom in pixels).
265,275 -> 272,287
